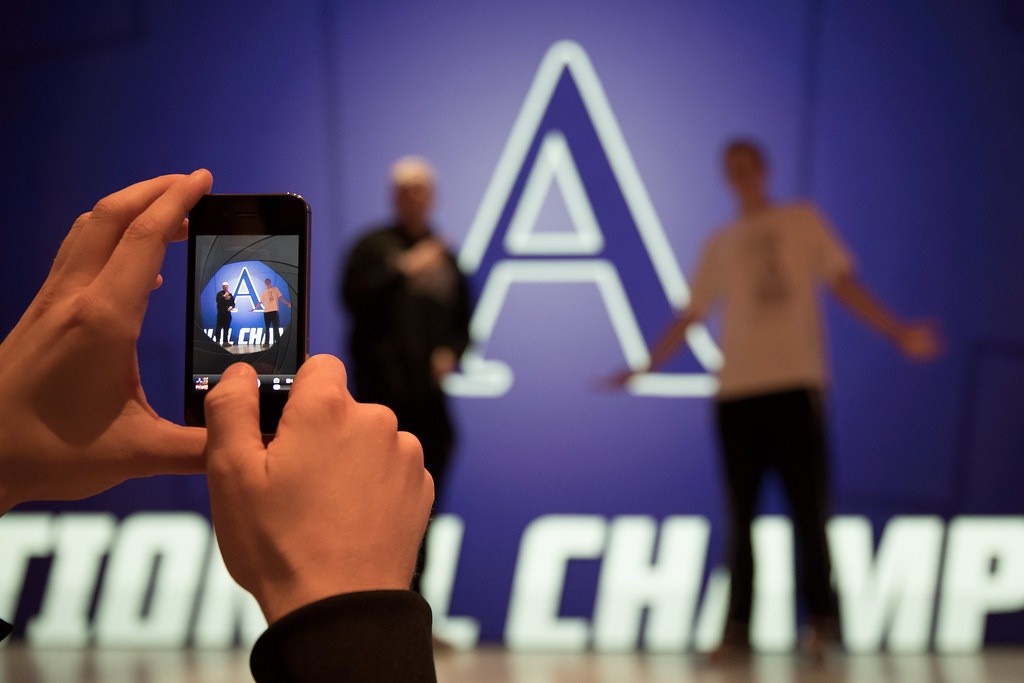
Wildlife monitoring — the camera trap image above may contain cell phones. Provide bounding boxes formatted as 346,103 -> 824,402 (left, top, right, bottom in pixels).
185,194 -> 312,437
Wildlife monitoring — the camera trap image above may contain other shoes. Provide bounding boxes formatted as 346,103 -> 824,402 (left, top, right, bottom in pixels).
263,343 -> 269,347
696,643 -> 752,666
223,342 -> 232,347
794,637 -> 842,662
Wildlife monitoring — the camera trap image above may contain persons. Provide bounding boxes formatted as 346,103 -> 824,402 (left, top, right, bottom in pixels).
216,282 -> 236,347
331,156 -> 478,657
598,140 -> 942,663
0,169 -> 437,683
249,279 -> 291,348
